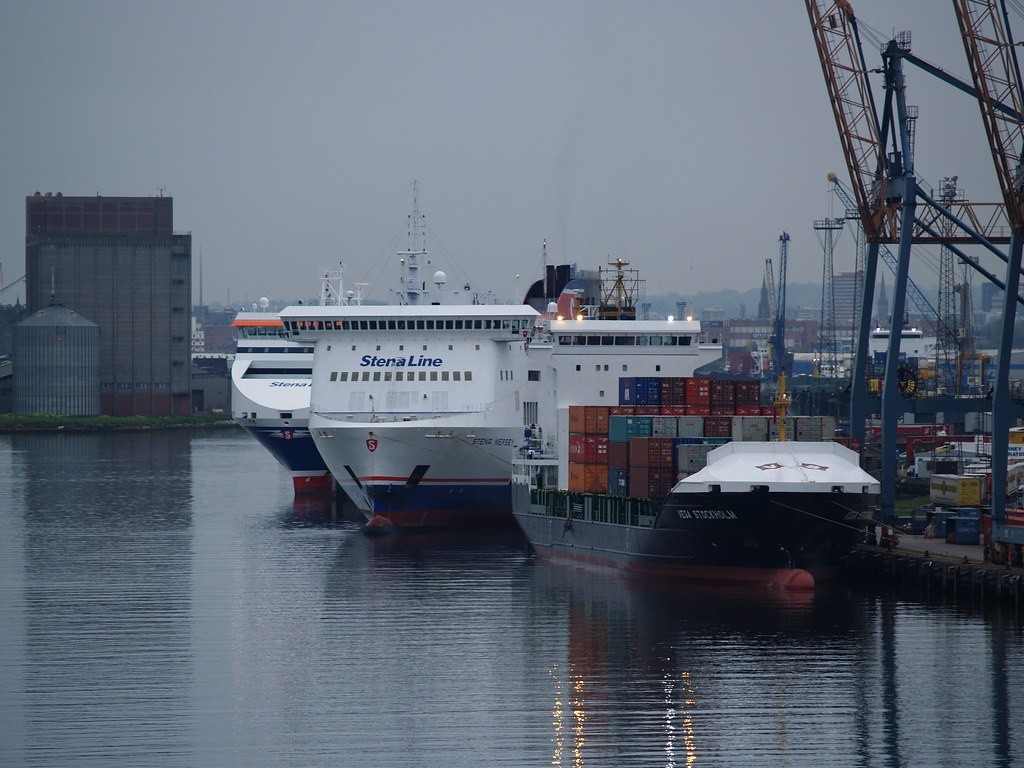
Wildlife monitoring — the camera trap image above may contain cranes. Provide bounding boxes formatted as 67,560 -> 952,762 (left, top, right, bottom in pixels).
827,173 -> 961,370
805,1 -> 1023,244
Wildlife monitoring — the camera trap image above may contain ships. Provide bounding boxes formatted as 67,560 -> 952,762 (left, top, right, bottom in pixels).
231,304 -> 882,590
